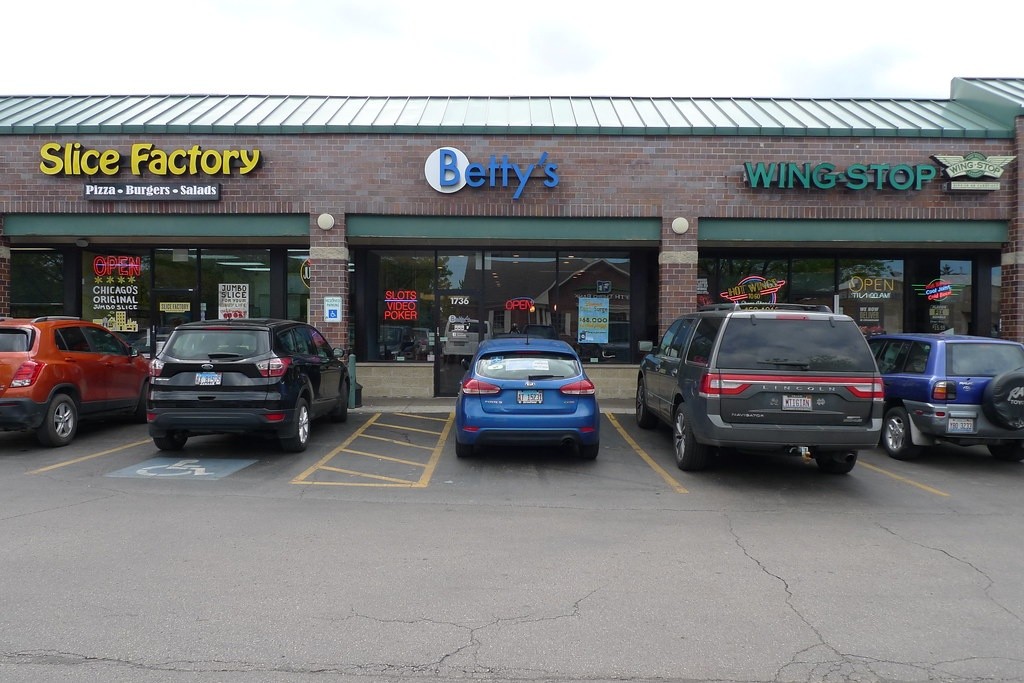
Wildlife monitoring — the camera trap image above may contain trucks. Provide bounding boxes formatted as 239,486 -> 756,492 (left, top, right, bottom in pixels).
440,317 -> 493,363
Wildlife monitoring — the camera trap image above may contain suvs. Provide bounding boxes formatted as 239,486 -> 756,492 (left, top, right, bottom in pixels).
0,316 -> 151,447
379,324 -> 419,358
854,332 -> 1024,463
145,317 -> 350,453
591,321 -> 630,361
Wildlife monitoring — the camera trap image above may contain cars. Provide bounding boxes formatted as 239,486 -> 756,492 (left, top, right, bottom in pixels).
523,323 -> 557,340
452,333 -> 601,461
414,326 -> 435,346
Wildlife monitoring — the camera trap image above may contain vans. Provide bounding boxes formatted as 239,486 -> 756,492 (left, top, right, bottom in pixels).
634,301 -> 886,476
